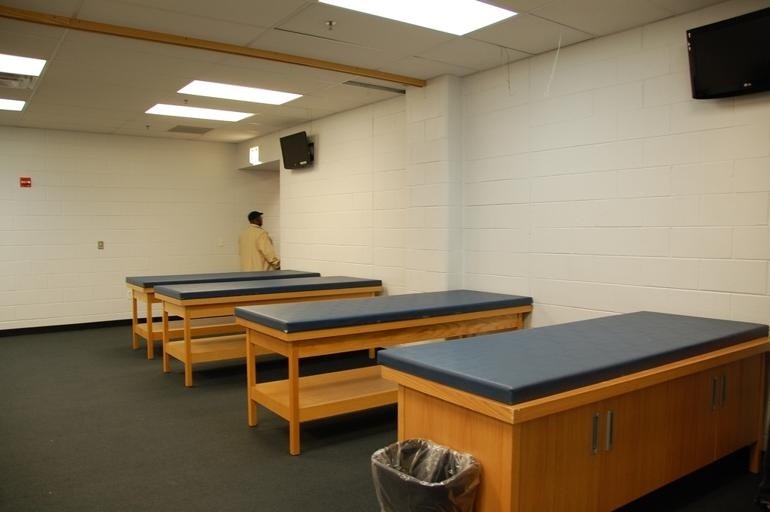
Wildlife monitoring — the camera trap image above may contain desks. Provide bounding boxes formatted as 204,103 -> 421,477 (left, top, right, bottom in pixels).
234,289 -> 532,455
127,270 -> 320,360
154,276 -> 382,387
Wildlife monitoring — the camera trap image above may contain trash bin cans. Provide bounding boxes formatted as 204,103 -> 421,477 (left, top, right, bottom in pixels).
371,438 -> 480,512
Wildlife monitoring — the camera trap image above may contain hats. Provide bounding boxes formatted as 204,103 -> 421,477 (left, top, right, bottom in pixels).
248,211 -> 263,221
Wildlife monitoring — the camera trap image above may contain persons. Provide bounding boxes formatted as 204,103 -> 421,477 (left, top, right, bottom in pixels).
237,210 -> 281,273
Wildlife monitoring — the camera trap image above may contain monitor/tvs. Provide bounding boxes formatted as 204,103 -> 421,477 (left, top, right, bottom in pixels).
686,7 -> 770,100
279,132 -> 314,169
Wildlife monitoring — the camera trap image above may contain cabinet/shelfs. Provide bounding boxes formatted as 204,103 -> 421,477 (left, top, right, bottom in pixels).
376,310 -> 770,512
